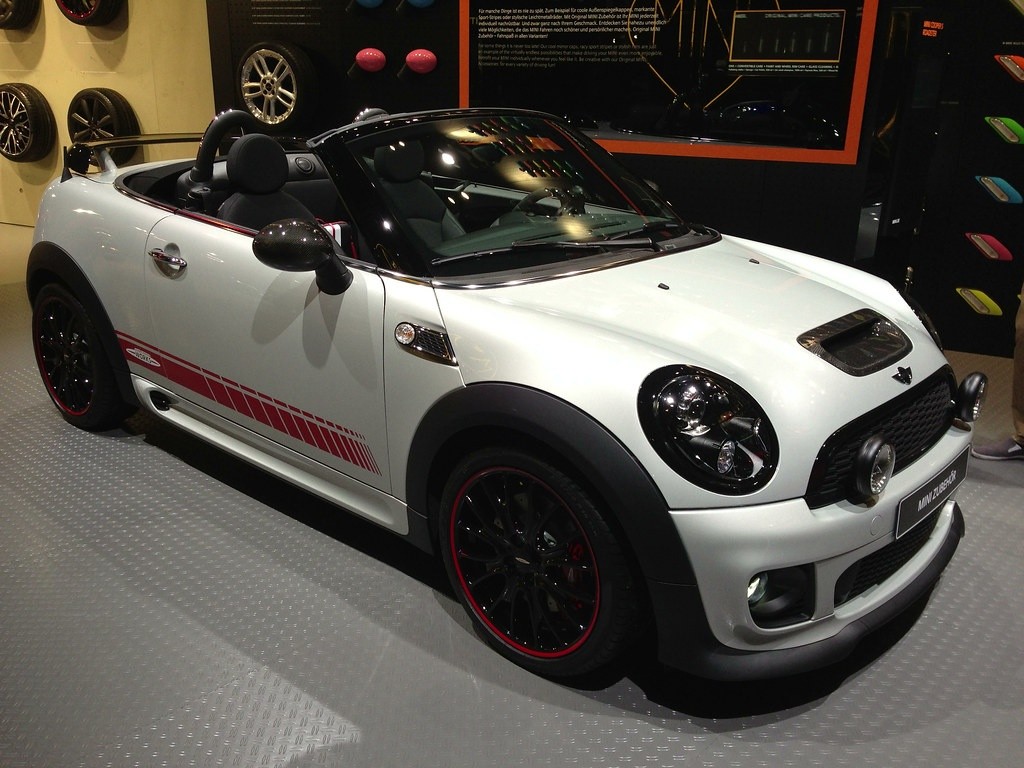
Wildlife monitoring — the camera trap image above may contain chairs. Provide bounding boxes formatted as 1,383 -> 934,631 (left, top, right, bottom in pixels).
217,133 -> 315,231
373,139 -> 467,248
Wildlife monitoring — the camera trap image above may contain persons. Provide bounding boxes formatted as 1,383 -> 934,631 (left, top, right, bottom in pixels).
971,283 -> 1024,459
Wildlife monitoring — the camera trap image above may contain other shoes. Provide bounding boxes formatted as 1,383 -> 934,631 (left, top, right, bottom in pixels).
970,438 -> 1024,460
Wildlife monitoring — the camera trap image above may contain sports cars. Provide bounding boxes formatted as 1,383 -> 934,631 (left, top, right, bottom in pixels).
24,102 -> 993,692
